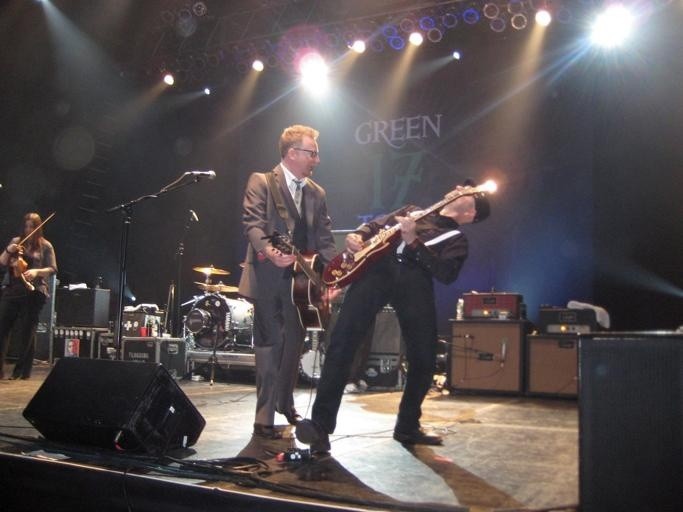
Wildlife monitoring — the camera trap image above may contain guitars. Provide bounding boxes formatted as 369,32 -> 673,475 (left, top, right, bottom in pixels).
271,232 -> 331,331
322,184 -> 481,289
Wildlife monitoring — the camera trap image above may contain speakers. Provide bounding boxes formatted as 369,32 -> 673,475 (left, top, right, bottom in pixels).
22,357 -> 206,456
575,331 -> 683,511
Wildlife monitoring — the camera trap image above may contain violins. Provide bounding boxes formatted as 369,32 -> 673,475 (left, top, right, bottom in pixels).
8,244 -> 36,294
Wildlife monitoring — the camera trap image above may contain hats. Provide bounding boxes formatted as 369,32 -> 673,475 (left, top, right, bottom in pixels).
464,179 -> 490,222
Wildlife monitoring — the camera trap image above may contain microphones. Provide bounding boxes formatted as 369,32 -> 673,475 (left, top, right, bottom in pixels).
190,170 -> 216,180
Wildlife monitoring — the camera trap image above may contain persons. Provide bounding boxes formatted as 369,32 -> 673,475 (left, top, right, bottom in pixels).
236,123 -> 342,439
1,211 -> 58,381
294,178 -> 491,457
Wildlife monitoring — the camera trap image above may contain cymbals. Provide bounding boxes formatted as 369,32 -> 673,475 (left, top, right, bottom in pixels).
192,267 -> 230,276
199,284 -> 241,294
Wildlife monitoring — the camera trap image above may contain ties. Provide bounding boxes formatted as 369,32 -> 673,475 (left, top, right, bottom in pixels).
293,179 -> 301,217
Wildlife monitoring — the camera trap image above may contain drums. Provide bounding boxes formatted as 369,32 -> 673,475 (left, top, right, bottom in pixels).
183,305 -> 211,338
189,296 -> 254,351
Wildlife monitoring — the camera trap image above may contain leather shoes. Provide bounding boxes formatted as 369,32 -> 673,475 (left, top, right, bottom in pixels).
254,406 -> 330,453
393,427 -> 442,443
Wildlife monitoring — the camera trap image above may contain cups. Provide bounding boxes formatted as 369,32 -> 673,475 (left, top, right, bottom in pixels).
140,327 -> 147,337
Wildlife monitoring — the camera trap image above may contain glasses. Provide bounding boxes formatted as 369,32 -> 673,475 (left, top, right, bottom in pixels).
293,149 -> 318,157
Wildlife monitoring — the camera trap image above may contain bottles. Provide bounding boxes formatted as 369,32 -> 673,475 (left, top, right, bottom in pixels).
145,319 -> 159,336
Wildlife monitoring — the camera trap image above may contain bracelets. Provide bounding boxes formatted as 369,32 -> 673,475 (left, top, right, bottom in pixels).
4,246 -> 13,256
